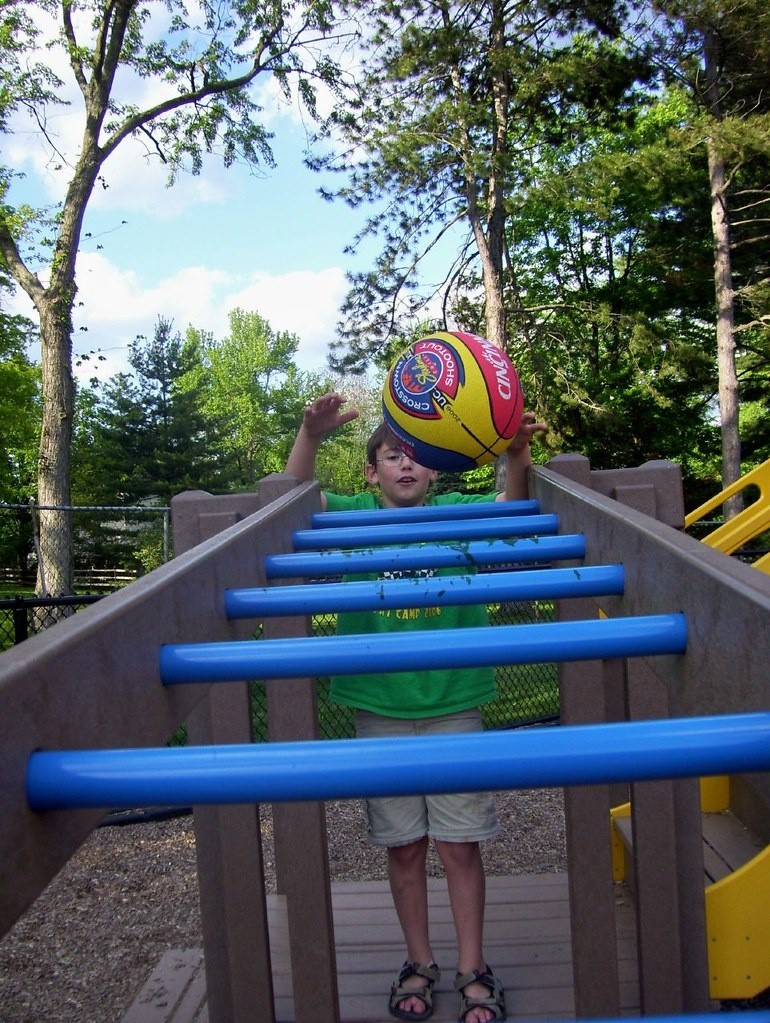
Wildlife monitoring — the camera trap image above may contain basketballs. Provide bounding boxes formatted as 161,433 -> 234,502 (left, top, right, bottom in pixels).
381,330 -> 523,473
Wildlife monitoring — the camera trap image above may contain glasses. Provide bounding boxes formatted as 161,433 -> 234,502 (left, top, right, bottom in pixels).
372,451 -> 408,467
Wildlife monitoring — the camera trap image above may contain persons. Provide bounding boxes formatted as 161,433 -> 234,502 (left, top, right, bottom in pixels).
284,392 -> 548,1022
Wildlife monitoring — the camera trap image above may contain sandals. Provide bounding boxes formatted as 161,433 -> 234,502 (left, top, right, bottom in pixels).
388,959 -> 440,1022
453,964 -> 507,1023
